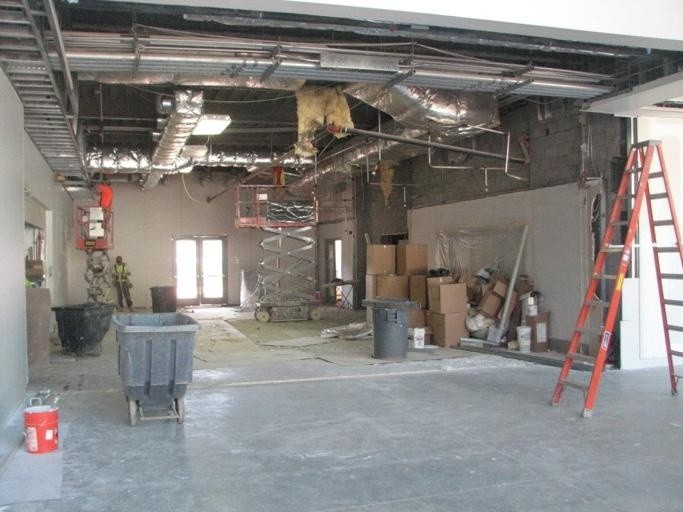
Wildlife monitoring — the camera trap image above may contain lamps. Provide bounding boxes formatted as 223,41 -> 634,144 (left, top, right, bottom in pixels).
191,115 -> 232,136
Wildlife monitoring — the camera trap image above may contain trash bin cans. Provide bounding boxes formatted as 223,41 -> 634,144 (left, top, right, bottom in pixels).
373,297 -> 409,360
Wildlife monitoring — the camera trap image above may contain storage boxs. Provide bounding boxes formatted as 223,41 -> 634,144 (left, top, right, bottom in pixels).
362,232 -> 550,354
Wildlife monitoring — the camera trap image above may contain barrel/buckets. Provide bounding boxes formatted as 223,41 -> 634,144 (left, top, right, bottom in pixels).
23,405 -> 61,455
517,324 -> 531,351
371,307 -> 408,359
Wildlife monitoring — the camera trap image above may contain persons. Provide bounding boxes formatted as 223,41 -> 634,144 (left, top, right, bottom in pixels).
111,255 -> 136,313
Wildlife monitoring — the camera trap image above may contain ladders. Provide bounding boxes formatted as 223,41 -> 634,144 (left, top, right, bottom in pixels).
149,286 -> 176,312
551,141 -> 683,418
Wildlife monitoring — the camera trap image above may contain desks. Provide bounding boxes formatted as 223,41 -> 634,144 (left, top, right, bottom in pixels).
320,280 -> 359,314
